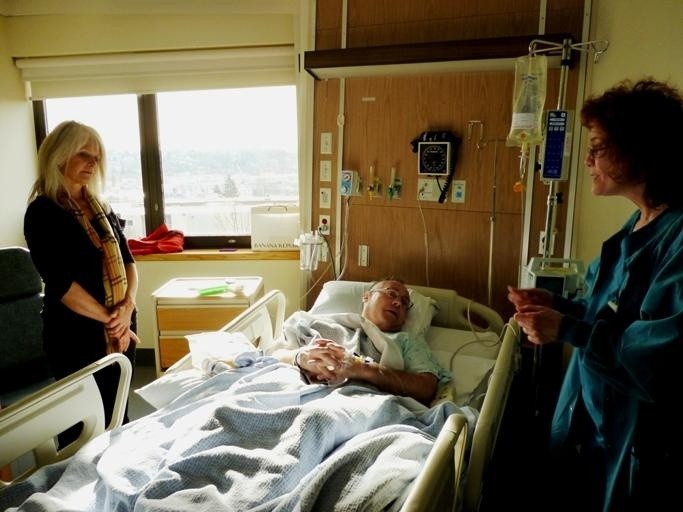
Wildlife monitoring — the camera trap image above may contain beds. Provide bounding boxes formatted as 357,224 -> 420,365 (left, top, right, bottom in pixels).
0,279 -> 526,512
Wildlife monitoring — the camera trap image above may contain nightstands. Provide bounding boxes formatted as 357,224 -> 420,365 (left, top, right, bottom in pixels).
151,277 -> 265,380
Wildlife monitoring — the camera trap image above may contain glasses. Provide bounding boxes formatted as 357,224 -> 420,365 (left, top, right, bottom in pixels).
586,143 -> 613,158
370,287 -> 414,312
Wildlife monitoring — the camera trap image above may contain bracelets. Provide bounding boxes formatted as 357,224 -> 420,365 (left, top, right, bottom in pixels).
289,348 -> 301,368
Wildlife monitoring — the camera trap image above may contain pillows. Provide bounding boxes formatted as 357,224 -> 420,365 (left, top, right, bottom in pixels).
307,281 -> 440,342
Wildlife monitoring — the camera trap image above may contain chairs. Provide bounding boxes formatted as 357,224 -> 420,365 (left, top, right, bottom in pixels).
0,248 -> 57,410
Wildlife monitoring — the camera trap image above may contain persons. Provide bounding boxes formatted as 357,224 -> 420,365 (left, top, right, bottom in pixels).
257,273 -> 455,399
505,73 -> 679,505
19,118 -> 142,441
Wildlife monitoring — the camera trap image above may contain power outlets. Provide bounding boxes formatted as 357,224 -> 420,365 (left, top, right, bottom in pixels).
319,215 -> 330,236
418,178 -> 435,201
